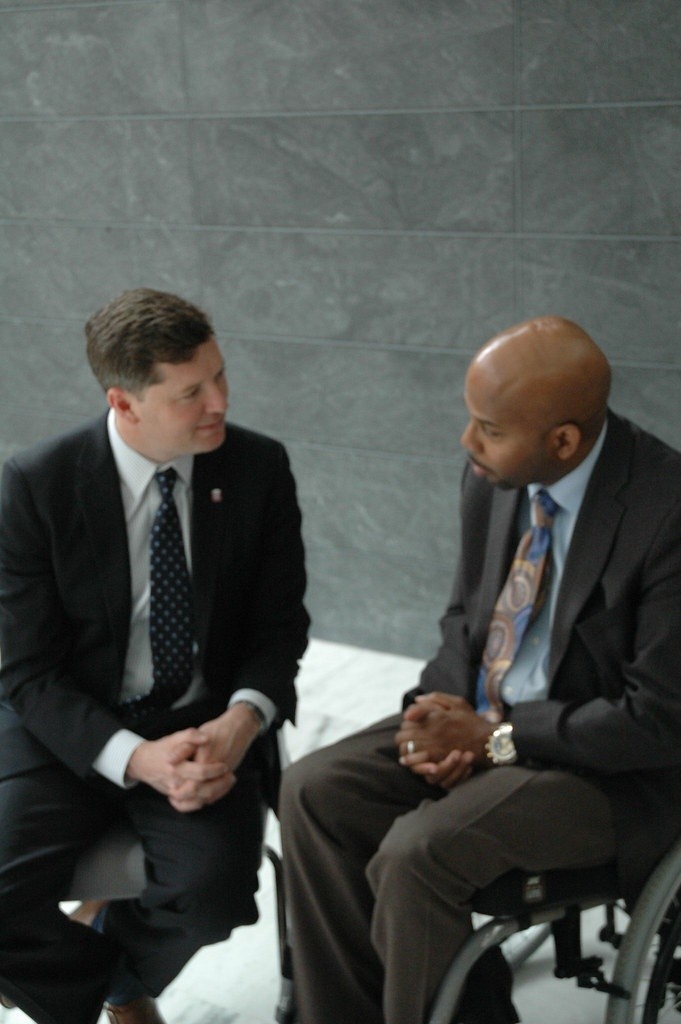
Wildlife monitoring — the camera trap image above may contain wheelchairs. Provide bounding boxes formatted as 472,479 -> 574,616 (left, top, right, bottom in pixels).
259,832 -> 681,1024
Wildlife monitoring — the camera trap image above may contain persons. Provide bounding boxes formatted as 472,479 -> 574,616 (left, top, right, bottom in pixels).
1,289 -> 312,1024
279,316 -> 681,1023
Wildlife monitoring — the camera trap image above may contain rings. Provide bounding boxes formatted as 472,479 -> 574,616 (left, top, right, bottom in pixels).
408,741 -> 414,753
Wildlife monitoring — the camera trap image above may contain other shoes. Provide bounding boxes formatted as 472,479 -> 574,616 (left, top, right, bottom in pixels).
105,998 -> 166,1024
1,897 -> 103,1010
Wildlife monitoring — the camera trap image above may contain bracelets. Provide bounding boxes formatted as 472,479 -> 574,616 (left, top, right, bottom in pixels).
242,701 -> 265,729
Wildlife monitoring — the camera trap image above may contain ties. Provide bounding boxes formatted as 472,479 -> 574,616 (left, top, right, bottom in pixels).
476,488 -> 559,724
124,468 -> 194,709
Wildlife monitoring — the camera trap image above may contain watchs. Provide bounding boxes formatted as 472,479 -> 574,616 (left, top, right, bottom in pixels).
484,722 -> 517,766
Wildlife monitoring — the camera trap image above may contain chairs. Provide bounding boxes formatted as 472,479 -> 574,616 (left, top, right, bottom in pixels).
57,732 -> 292,966
